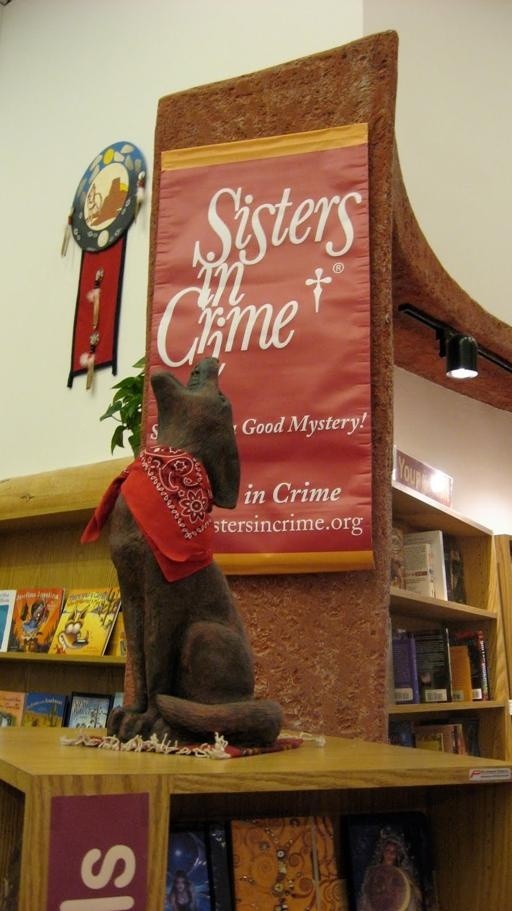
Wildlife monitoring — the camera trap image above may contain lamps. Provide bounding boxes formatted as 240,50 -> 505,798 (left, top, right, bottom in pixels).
434,327 -> 481,382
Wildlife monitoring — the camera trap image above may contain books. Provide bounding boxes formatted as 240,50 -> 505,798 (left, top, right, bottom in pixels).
390,524 -> 492,758
0,584 -> 131,732
165,810 -> 442,911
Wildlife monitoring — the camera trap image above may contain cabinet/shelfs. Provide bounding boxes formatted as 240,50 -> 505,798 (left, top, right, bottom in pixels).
2,452 -> 128,731
392,477 -> 512,761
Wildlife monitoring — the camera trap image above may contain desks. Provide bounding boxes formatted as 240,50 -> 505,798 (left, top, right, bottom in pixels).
0,727 -> 510,911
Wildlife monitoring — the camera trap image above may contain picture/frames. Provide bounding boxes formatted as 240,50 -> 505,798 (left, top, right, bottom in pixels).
168,807 -> 439,910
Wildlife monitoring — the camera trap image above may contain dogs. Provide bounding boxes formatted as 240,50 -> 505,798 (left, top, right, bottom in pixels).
89,351 -> 285,747
